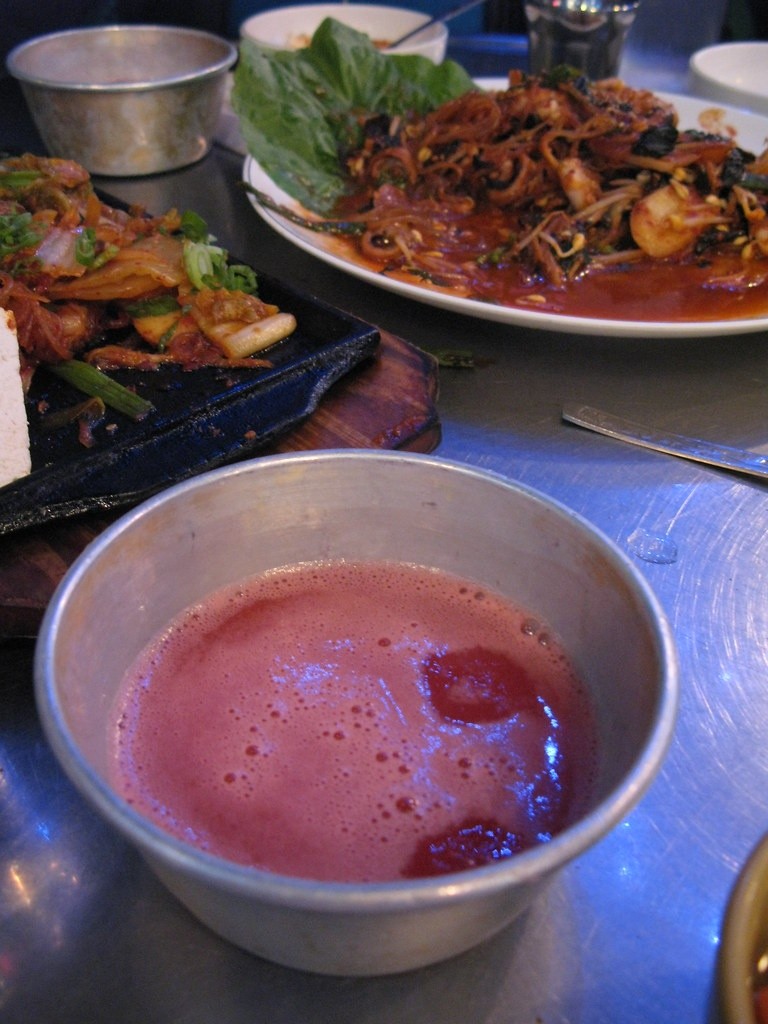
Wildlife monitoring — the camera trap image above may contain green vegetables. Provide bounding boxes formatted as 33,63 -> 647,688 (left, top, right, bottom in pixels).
52,357 -> 157,420
227,16 -> 486,217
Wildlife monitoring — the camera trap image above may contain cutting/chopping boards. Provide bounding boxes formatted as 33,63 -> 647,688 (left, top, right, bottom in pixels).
1,295 -> 443,636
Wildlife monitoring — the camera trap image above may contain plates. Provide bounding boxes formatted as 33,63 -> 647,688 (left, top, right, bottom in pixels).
243,79 -> 767,337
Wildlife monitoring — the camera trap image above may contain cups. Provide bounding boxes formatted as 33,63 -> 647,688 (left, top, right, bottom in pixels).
33,448 -> 678,978
5,25 -> 239,175
523,1 -> 639,80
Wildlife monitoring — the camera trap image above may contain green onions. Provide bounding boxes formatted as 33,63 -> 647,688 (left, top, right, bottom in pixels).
0,167 -> 259,352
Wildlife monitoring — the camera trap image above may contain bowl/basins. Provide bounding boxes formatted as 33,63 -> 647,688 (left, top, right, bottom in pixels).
242,5 -> 450,69
691,43 -> 766,112
